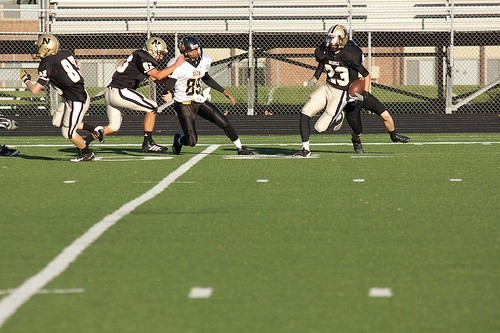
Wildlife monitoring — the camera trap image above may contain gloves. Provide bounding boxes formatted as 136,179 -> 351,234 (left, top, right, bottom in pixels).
20,70 -> 30,84
349,92 -> 368,102
307,78 -> 317,88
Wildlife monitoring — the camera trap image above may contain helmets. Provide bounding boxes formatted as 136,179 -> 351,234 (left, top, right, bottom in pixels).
326,24 -> 349,52
143,36 -> 169,62
178,35 -> 203,64
36,34 -> 59,59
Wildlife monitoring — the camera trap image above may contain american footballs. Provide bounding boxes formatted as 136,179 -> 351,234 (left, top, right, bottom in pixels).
348,78 -> 365,97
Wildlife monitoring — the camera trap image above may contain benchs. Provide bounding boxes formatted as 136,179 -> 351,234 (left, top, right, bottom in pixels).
42,2 -> 500,34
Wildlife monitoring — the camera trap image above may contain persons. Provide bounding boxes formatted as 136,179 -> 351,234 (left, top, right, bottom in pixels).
293,22 -> 370,156
162,36 -> 255,154
83,37 -> 185,152
343,54 -> 410,150
20,35 -> 105,161
0,118 -> 21,156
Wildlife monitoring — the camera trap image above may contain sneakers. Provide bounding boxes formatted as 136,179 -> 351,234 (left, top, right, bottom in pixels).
0,144 -> 18,156
351,136 -> 365,154
333,110 -> 345,131
391,134 -> 410,143
293,147 -> 312,157
71,151 -> 94,162
236,145 -> 255,155
171,133 -> 182,155
94,125 -> 105,144
142,139 -> 168,153
